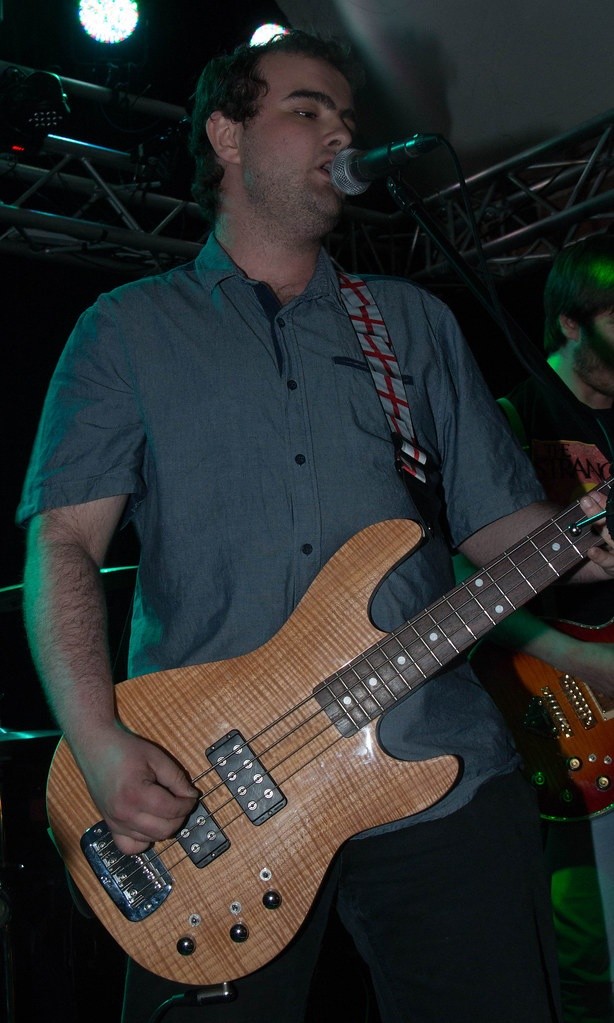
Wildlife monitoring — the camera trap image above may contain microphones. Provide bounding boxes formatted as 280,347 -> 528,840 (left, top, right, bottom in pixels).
330,132 -> 444,197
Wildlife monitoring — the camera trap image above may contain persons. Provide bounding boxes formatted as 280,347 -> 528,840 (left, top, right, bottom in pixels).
454,229 -> 614,1023
18,29 -> 614,1022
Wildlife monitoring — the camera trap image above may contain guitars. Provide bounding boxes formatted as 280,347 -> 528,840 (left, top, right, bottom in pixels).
43,460 -> 614,986
464,606 -> 614,824
